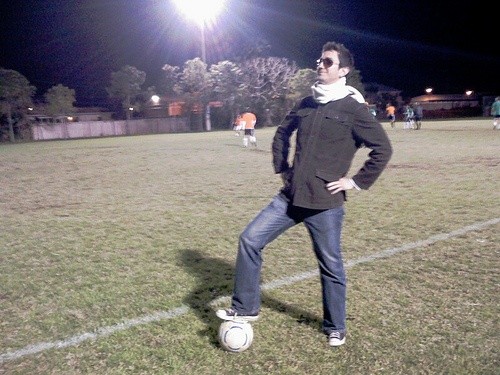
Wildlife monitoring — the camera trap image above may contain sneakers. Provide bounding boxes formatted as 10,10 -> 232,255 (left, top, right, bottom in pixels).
329,330 -> 345,347
215,308 -> 258,320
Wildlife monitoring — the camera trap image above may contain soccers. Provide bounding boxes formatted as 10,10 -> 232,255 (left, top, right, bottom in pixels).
216,318 -> 254,353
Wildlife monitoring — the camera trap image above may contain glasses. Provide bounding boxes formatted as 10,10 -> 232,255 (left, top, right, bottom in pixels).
315,57 -> 339,67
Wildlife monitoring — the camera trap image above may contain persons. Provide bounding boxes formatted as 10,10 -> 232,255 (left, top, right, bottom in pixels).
406,106 -> 414,128
234,113 -> 241,136
216,41 -> 394,346
491,97 -> 500,128
385,104 -> 395,128
240,107 -> 256,149
414,102 -> 423,129
